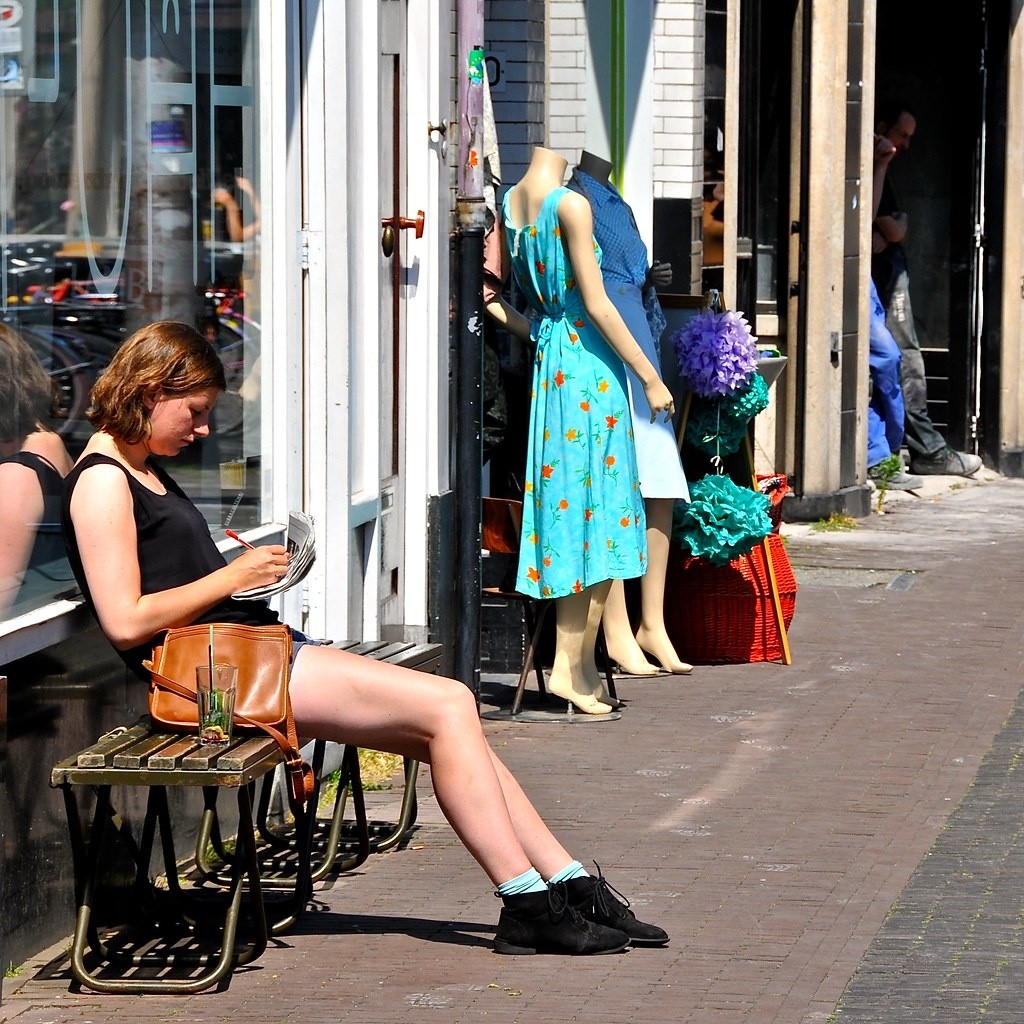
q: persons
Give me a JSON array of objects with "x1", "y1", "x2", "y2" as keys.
[
  {"x1": 875, "y1": 108, "x2": 982, "y2": 476},
  {"x1": 566, "y1": 151, "x2": 693, "y2": 676},
  {"x1": 61, "y1": 320, "x2": 670, "y2": 957},
  {"x1": 502, "y1": 147, "x2": 675, "y2": 715},
  {"x1": 0, "y1": 325, "x2": 73, "y2": 857}
]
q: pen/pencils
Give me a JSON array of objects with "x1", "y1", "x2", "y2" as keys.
[{"x1": 226, "y1": 529, "x2": 256, "y2": 552}]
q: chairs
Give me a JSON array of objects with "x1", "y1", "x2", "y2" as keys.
[{"x1": 479, "y1": 496, "x2": 618, "y2": 716}]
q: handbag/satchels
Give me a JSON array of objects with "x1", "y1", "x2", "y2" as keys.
[{"x1": 140, "y1": 621, "x2": 292, "y2": 732}]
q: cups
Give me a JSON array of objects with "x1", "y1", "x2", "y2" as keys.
[
  {"x1": 232, "y1": 458, "x2": 246, "y2": 489},
  {"x1": 196, "y1": 665, "x2": 238, "y2": 747},
  {"x1": 219, "y1": 460, "x2": 243, "y2": 489}
]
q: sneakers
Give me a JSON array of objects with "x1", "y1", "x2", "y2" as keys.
[
  {"x1": 911, "y1": 444, "x2": 982, "y2": 475},
  {"x1": 556, "y1": 862, "x2": 672, "y2": 944},
  {"x1": 491, "y1": 891, "x2": 630, "y2": 957},
  {"x1": 867, "y1": 466, "x2": 924, "y2": 490}
]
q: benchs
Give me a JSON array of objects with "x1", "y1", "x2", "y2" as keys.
[{"x1": 50, "y1": 640, "x2": 447, "y2": 993}]
